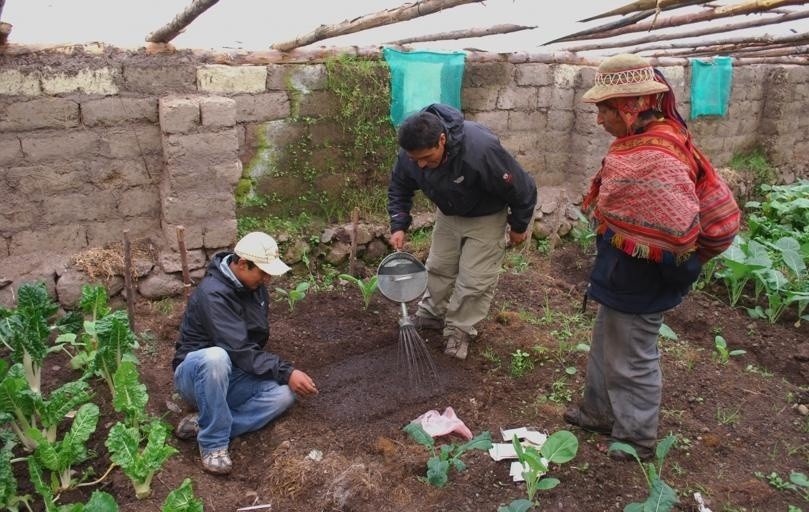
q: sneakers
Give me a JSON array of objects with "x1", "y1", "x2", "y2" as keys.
[
  {"x1": 198, "y1": 443, "x2": 232, "y2": 474},
  {"x1": 176, "y1": 413, "x2": 200, "y2": 439},
  {"x1": 410, "y1": 314, "x2": 468, "y2": 359},
  {"x1": 611, "y1": 446, "x2": 653, "y2": 462},
  {"x1": 565, "y1": 410, "x2": 610, "y2": 430}
]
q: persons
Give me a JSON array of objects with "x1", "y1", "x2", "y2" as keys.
[
  {"x1": 387, "y1": 112, "x2": 536, "y2": 361},
  {"x1": 563, "y1": 52, "x2": 742, "y2": 462},
  {"x1": 171, "y1": 231, "x2": 320, "y2": 475}
]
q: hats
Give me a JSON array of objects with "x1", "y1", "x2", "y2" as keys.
[
  {"x1": 583, "y1": 55, "x2": 670, "y2": 102},
  {"x1": 234, "y1": 232, "x2": 292, "y2": 276}
]
